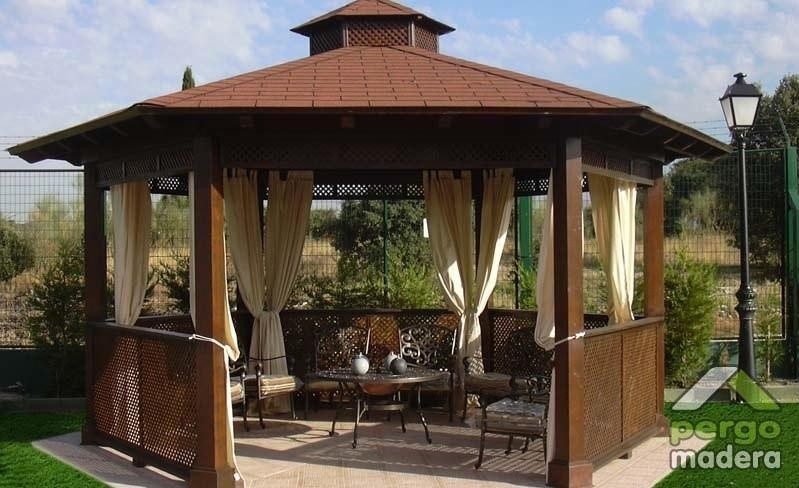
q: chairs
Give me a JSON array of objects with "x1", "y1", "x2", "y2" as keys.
[{"x1": 167, "y1": 324, "x2": 554, "y2": 469}]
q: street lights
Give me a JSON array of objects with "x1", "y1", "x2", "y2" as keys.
[{"x1": 719, "y1": 71, "x2": 763, "y2": 402}]
